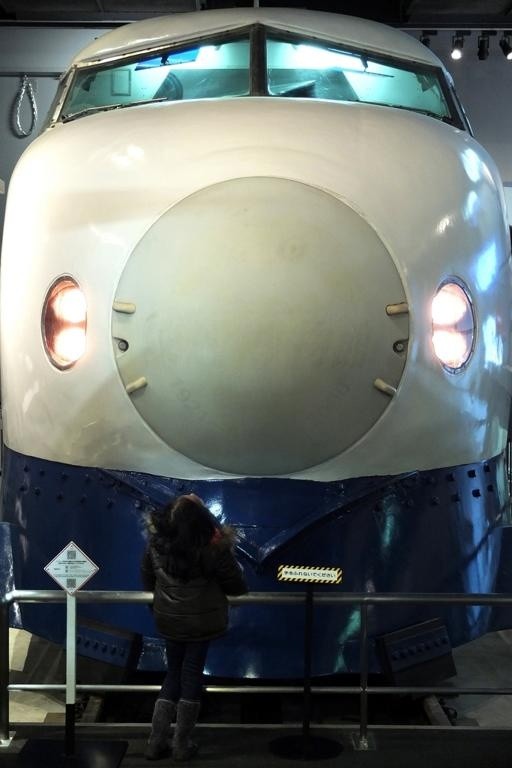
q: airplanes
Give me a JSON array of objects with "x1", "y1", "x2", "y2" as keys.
[{"x1": 0, "y1": 2, "x2": 511, "y2": 726}]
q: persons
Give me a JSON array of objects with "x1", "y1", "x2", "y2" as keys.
[{"x1": 141, "y1": 492, "x2": 250, "y2": 761}]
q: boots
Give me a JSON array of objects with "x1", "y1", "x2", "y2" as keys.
[
  {"x1": 174, "y1": 698, "x2": 200, "y2": 761},
  {"x1": 145, "y1": 699, "x2": 176, "y2": 758}
]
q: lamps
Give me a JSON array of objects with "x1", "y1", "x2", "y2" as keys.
[{"x1": 418, "y1": 28, "x2": 512, "y2": 62}]
